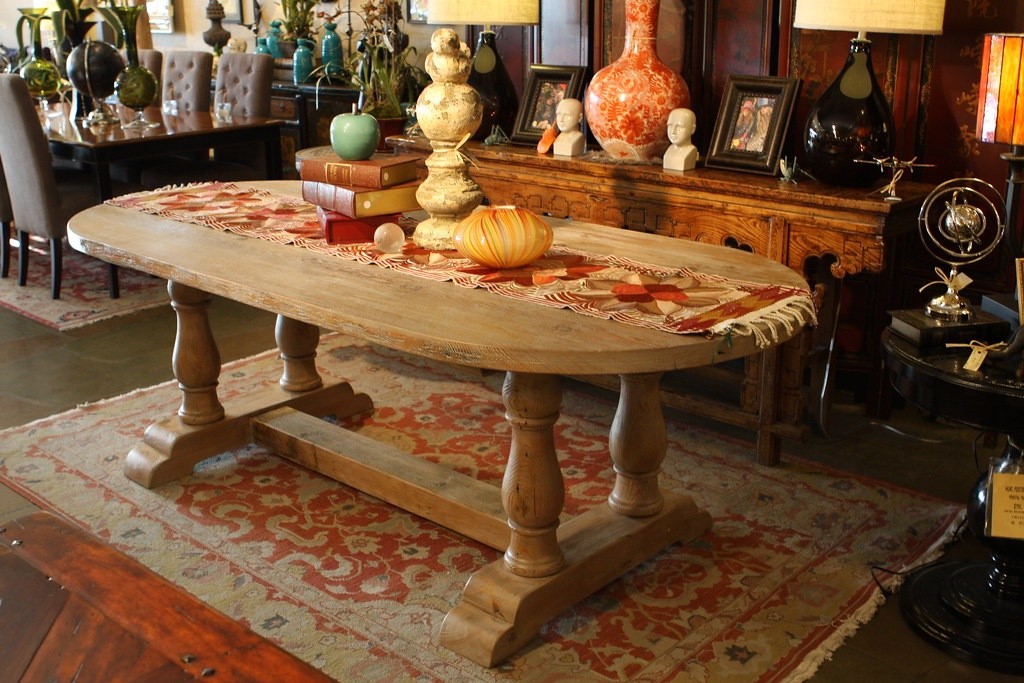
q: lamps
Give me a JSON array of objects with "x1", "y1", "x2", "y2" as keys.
[
  {"x1": 791, "y1": 0, "x2": 947, "y2": 192},
  {"x1": 427, "y1": 0, "x2": 540, "y2": 140},
  {"x1": 976, "y1": 34, "x2": 1024, "y2": 293}
]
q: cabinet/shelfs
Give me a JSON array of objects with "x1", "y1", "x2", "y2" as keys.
[{"x1": 210, "y1": 78, "x2": 360, "y2": 180}]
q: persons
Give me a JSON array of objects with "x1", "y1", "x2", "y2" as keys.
[
  {"x1": 535, "y1": 90, "x2": 557, "y2": 130},
  {"x1": 730, "y1": 99, "x2": 773, "y2": 151},
  {"x1": 552, "y1": 99, "x2": 586, "y2": 156},
  {"x1": 662, "y1": 107, "x2": 698, "y2": 171}
]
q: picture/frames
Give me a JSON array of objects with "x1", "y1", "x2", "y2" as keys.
[
  {"x1": 705, "y1": 74, "x2": 804, "y2": 178},
  {"x1": 218, "y1": 0, "x2": 244, "y2": 25},
  {"x1": 509, "y1": 63, "x2": 589, "y2": 151},
  {"x1": 406, "y1": 0, "x2": 456, "y2": 27}
]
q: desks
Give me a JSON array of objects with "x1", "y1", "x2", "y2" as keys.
[
  {"x1": 878, "y1": 303, "x2": 1024, "y2": 676},
  {"x1": 385, "y1": 129, "x2": 937, "y2": 466},
  {"x1": 67, "y1": 177, "x2": 816, "y2": 663}
]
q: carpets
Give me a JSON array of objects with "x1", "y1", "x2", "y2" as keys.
[
  {"x1": 0, "y1": 174, "x2": 171, "y2": 333},
  {"x1": 0, "y1": 336, "x2": 969, "y2": 683}
]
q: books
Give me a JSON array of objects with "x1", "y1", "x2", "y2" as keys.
[{"x1": 296, "y1": 148, "x2": 424, "y2": 245}]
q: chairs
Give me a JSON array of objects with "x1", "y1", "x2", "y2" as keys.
[{"x1": 0, "y1": 46, "x2": 274, "y2": 299}]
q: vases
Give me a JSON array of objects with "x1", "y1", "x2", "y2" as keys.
[
  {"x1": 268, "y1": 20, "x2": 286, "y2": 58},
  {"x1": 293, "y1": 38, "x2": 315, "y2": 86},
  {"x1": 17, "y1": 5, "x2": 61, "y2": 118},
  {"x1": 584, "y1": 0, "x2": 692, "y2": 165},
  {"x1": 93, "y1": 5, "x2": 127, "y2": 51},
  {"x1": 61, "y1": 20, "x2": 99, "y2": 122},
  {"x1": 320, "y1": 25, "x2": 343, "y2": 76},
  {"x1": 111, "y1": 3, "x2": 160, "y2": 130},
  {"x1": 255, "y1": 38, "x2": 272, "y2": 55}
]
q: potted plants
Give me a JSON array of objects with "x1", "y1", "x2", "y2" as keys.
[
  {"x1": 276, "y1": 0, "x2": 325, "y2": 57},
  {"x1": 307, "y1": 38, "x2": 427, "y2": 154}
]
